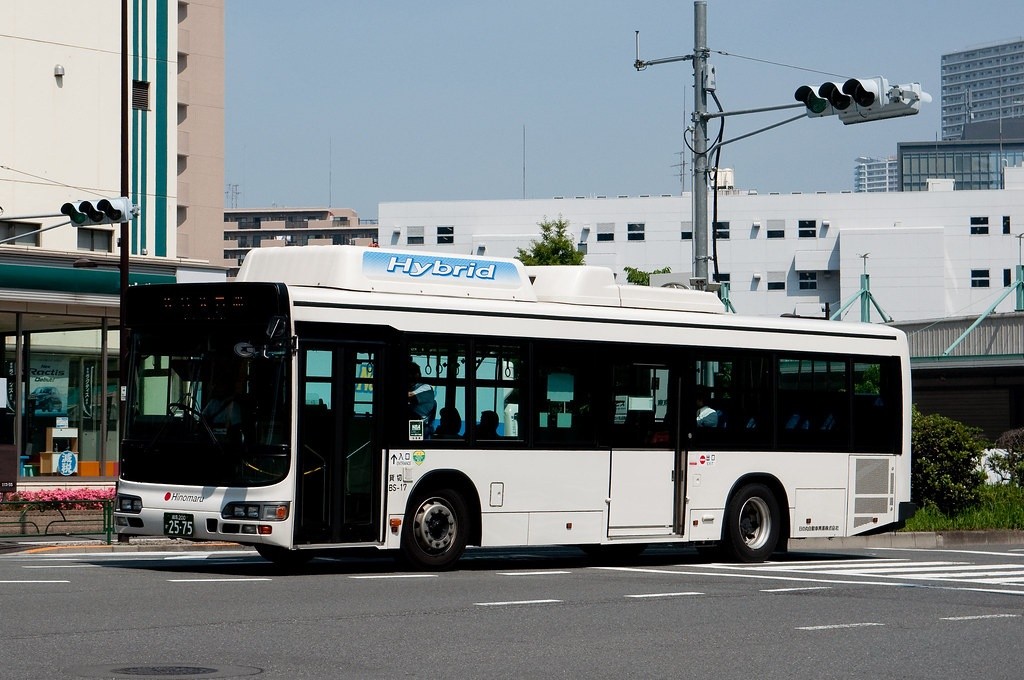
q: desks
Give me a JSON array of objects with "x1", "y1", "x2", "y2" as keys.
[
  {"x1": 19, "y1": 456, "x2": 29, "y2": 477},
  {"x1": 39, "y1": 452, "x2": 78, "y2": 477}
]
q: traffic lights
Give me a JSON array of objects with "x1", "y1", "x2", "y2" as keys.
[
  {"x1": 61, "y1": 196, "x2": 131, "y2": 228},
  {"x1": 792, "y1": 75, "x2": 889, "y2": 118},
  {"x1": 837, "y1": 81, "x2": 933, "y2": 125}
]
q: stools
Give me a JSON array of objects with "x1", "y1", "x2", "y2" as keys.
[{"x1": 24, "y1": 465, "x2": 34, "y2": 477}]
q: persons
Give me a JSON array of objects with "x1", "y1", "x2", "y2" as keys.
[
  {"x1": 202, "y1": 385, "x2": 233, "y2": 430},
  {"x1": 407, "y1": 361, "x2": 434, "y2": 419},
  {"x1": 696, "y1": 384, "x2": 718, "y2": 427},
  {"x1": 436, "y1": 408, "x2": 461, "y2": 437},
  {"x1": 475, "y1": 411, "x2": 499, "y2": 437}
]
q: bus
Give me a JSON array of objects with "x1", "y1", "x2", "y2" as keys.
[{"x1": 113, "y1": 241, "x2": 916, "y2": 574}]
